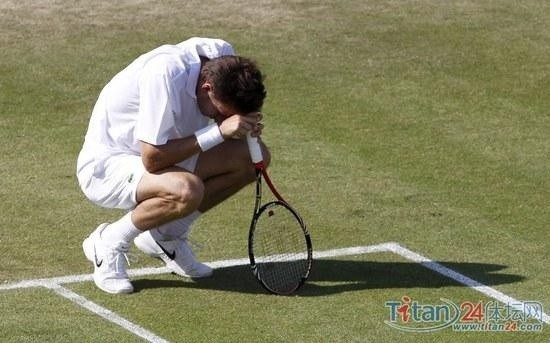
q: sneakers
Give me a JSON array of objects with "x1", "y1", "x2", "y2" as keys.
[
  {"x1": 83, "y1": 223, "x2": 134, "y2": 293},
  {"x1": 133, "y1": 229, "x2": 212, "y2": 277}
]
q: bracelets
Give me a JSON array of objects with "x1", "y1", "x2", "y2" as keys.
[{"x1": 193, "y1": 122, "x2": 225, "y2": 152}]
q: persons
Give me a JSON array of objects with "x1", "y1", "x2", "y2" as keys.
[{"x1": 75, "y1": 35, "x2": 268, "y2": 295}]
